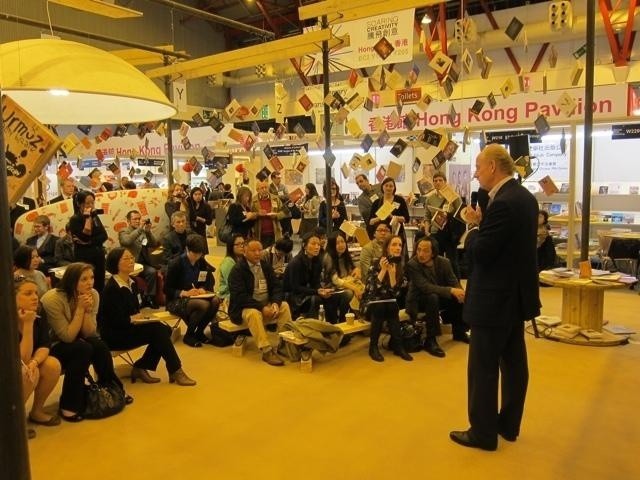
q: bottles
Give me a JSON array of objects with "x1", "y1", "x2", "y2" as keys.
[{"x1": 318, "y1": 305, "x2": 326, "y2": 323}]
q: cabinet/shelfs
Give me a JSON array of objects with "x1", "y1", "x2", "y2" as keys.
[{"x1": 537, "y1": 192, "x2": 640, "y2": 281}]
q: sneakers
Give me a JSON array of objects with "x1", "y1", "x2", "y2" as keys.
[
  {"x1": 184, "y1": 333, "x2": 212, "y2": 347},
  {"x1": 28, "y1": 412, "x2": 61, "y2": 426}
]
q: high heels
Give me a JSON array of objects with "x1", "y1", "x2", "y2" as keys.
[
  {"x1": 131, "y1": 364, "x2": 160, "y2": 383},
  {"x1": 169, "y1": 367, "x2": 196, "y2": 386}
]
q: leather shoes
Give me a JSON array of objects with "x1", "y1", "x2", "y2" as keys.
[
  {"x1": 59, "y1": 407, "x2": 82, "y2": 422},
  {"x1": 424, "y1": 337, "x2": 446, "y2": 357},
  {"x1": 262, "y1": 351, "x2": 283, "y2": 364},
  {"x1": 450, "y1": 430, "x2": 497, "y2": 450},
  {"x1": 454, "y1": 330, "x2": 470, "y2": 343},
  {"x1": 143, "y1": 294, "x2": 160, "y2": 309}
]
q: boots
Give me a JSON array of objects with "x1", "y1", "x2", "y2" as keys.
[
  {"x1": 370, "y1": 315, "x2": 385, "y2": 361},
  {"x1": 387, "y1": 315, "x2": 412, "y2": 360}
]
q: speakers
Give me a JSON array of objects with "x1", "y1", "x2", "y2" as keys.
[{"x1": 509, "y1": 135, "x2": 530, "y2": 161}]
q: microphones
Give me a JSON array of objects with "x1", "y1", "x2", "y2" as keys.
[{"x1": 471, "y1": 191, "x2": 479, "y2": 211}]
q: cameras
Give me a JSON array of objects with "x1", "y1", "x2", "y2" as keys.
[
  {"x1": 176, "y1": 191, "x2": 187, "y2": 198},
  {"x1": 388, "y1": 255, "x2": 402, "y2": 264},
  {"x1": 91, "y1": 208, "x2": 105, "y2": 218}
]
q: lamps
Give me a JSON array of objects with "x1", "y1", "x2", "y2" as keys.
[{"x1": 0, "y1": 1, "x2": 178, "y2": 127}]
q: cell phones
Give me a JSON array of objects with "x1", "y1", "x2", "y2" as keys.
[{"x1": 145, "y1": 219, "x2": 150, "y2": 225}]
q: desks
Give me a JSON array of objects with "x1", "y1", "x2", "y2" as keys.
[{"x1": 535, "y1": 267, "x2": 634, "y2": 347}]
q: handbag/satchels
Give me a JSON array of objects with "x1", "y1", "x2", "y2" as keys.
[
  {"x1": 84, "y1": 380, "x2": 127, "y2": 419},
  {"x1": 210, "y1": 318, "x2": 235, "y2": 346},
  {"x1": 360, "y1": 284, "x2": 399, "y2": 316},
  {"x1": 389, "y1": 320, "x2": 425, "y2": 353},
  {"x1": 219, "y1": 220, "x2": 242, "y2": 242}
]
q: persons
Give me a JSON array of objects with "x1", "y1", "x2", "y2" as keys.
[{"x1": 6, "y1": 143, "x2": 567, "y2": 454}]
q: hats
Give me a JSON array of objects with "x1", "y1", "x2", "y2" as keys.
[{"x1": 186, "y1": 234, "x2": 209, "y2": 256}]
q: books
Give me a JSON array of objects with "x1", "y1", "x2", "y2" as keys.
[
  {"x1": 536, "y1": 259, "x2": 639, "y2": 342},
  {"x1": 1, "y1": 92, "x2": 65, "y2": 207},
  {"x1": 60, "y1": 16, "x2": 639, "y2": 245}
]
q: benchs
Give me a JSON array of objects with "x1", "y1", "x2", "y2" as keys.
[
  {"x1": 110, "y1": 344, "x2": 142, "y2": 367},
  {"x1": 278, "y1": 311, "x2": 411, "y2": 346},
  {"x1": 130, "y1": 311, "x2": 183, "y2": 329},
  {"x1": 218, "y1": 319, "x2": 278, "y2": 333}
]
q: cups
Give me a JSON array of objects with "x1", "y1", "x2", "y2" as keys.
[{"x1": 345, "y1": 312, "x2": 356, "y2": 327}]
